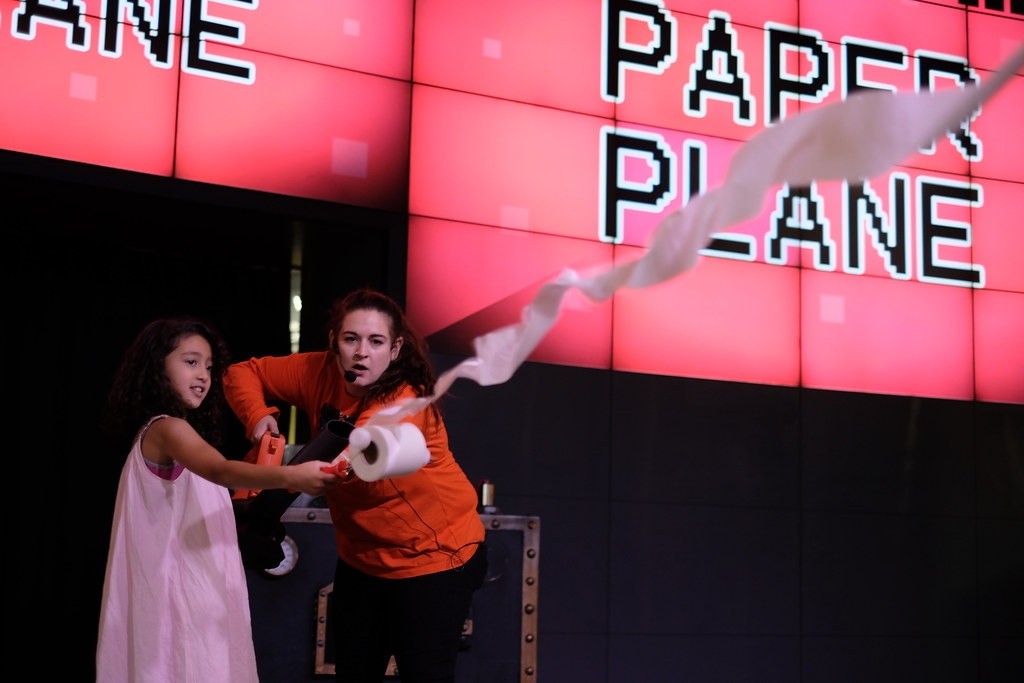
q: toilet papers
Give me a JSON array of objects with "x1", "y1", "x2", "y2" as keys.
[{"x1": 349, "y1": 45, "x2": 1024, "y2": 482}]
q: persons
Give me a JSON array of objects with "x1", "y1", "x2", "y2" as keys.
[
  {"x1": 95, "y1": 319, "x2": 342, "y2": 683},
  {"x1": 220, "y1": 290, "x2": 488, "y2": 683}
]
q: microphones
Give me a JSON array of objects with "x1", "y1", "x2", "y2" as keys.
[{"x1": 332, "y1": 337, "x2": 357, "y2": 383}]
